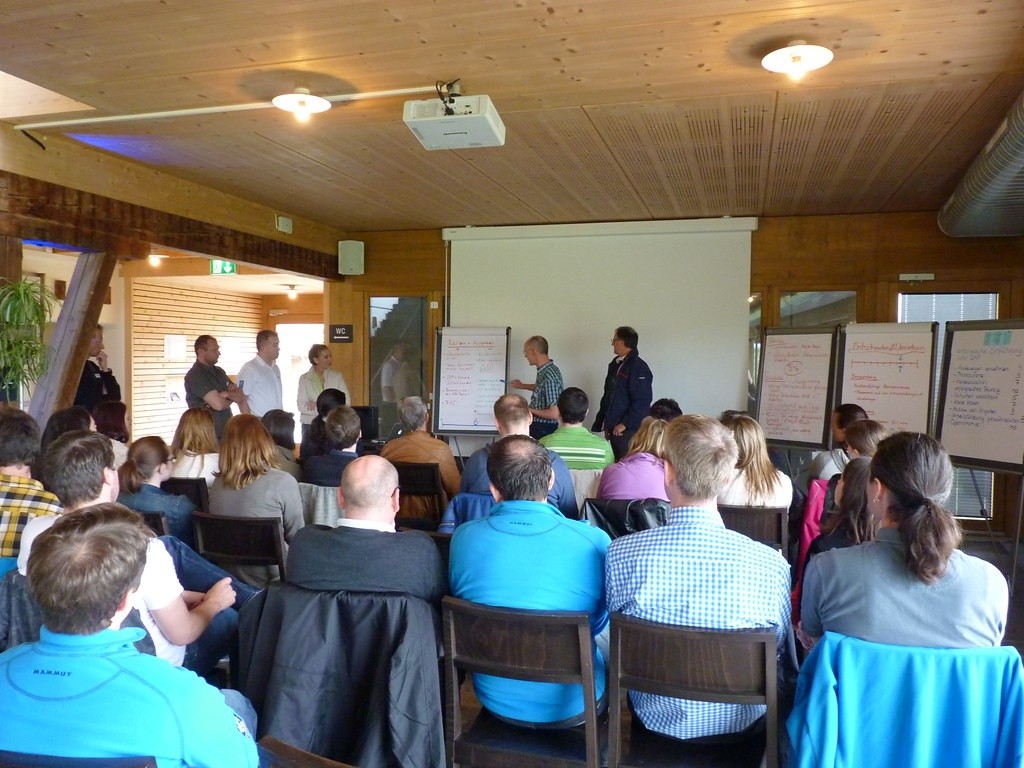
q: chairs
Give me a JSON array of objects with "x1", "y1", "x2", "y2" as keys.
[{"x1": 133, "y1": 454, "x2": 1024, "y2": 768}]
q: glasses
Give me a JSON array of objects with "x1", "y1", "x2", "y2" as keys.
[
  {"x1": 523, "y1": 351, "x2": 533, "y2": 354},
  {"x1": 166, "y1": 457, "x2": 176, "y2": 464},
  {"x1": 611, "y1": 339, "x2": 622, "y2": 342}
]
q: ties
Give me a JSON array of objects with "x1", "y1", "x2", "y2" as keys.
[{"x1": 612, "y1": 360, "x2": 622, "y2": 380}]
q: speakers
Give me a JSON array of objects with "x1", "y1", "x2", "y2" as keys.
[{"x1": 337, "y1": 240, "x2": 364, "y2": 275}]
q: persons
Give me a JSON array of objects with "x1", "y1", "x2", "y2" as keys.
[
  {"x1": 73, "y1": 324, "x2": 120, "y2": 412},
  {"x1": 379, "y1": 340, "x2": 407, "y2": 438},
  {"x1": 299, "y1": 344, "x2": 351, "y2": 425},
  {"x1": 392, "y1": 347, "x2": 423, "y2": 400},
  {"x1": 93, "y1": 400, "x2": 131, "y2": 468},
  {"x1": 0, "y1": 503, "x2": 258, "y2": 768},
  {"x1": 20, "y1": 430, "x2": 267, "y2": 677},
  {"x1": 169, "y1": 408, "x2": 220, "y2": 497},
  {"x1": 798, "y1": 432, "x2": 1008, "y2": 658},
  {"x1": 238, "y1": 330, "x2": 284, "y2": 422},
  {"x1": 308, "y1": 326, "x2": 886, "y2": 637},
  {"x1": 184, "y1": 336, "x2": 251, "y2": 437},
  {"x1": 261, "y1": 408, "x2": 304, "y2": 482},
  {"x1": 207, "y1": 415, "x2": 306, "y2": 587},
  {"x1": 300, "y1": 388, "x2": 347, "y2": 464},
  {"x1": 449, "y1": 435, "x2": 610, "y2": 732},
  {"x1": 41, "y1": 407, "x2": 98, "y2": 451},
  {"x1": 599, "y1": 416, "x2": 795, "y2": 768},
  {"x1": 115, "y1": 435, "x2": 198, "y2": 550},
  {"x1": 0, "y1": 400, "x2": 62, "y2": 557},
  {"x1": 336, "y1": 455, "x2": 466, "y2": 688}
]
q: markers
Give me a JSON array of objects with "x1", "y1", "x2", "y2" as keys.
[{"x1": 500, "y1": 380, "x2": 510, "y2": 384}]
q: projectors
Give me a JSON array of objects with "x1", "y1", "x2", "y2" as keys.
[{"x1": 402, "y1": 94, "x2": 506, "y2": 150}]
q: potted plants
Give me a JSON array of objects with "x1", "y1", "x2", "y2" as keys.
[{"x1": 0, "y1": 271, "x2": 65, "y2": 408}]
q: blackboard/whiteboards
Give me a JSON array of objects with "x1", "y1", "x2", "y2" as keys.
[
  {"x1": 838, "y1": 321, "x2": 940, "y2": 440},
  {"x1": 431, "y1": 326, "x2": 512, "y2": 436},
  {"x1": 756, "y1": 326, "x2": 841, "y2": 451},
  {"x1": 935, "y1": 318, "x2": 1024, "y2": 475}
]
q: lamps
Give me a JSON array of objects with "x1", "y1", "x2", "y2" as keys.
[
  {"x1": 760, "y1": 39, "x2": 834, "y2": 74},
  {"x1": 271, "y1": 88, "x2": 333, "y2": 120}
]
